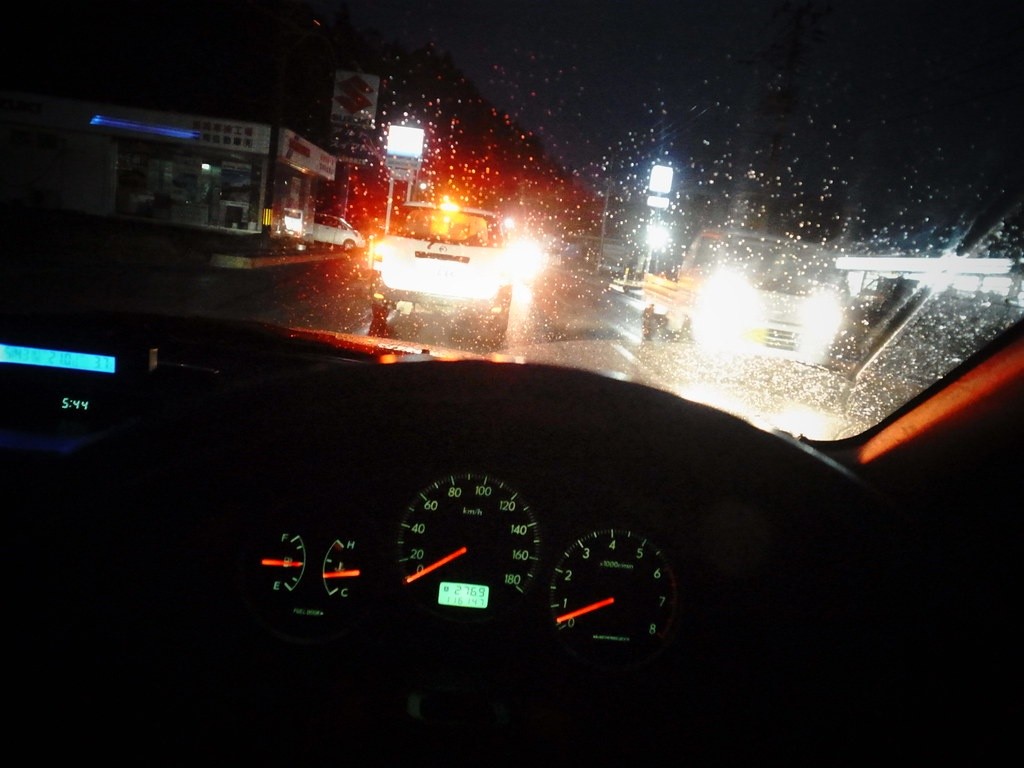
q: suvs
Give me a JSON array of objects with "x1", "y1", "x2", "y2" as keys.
[{"x1": 372, "y1": 199, "x2": 515, "y2": 335}]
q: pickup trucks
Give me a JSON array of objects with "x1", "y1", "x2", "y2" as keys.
[{"x1": 642, "y1": 229, "x2": 851, "y2": 373}]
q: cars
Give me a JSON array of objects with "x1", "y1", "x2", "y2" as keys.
[{"x1": 282, "y1": 207, "x2": 366, "y2": 253}]
